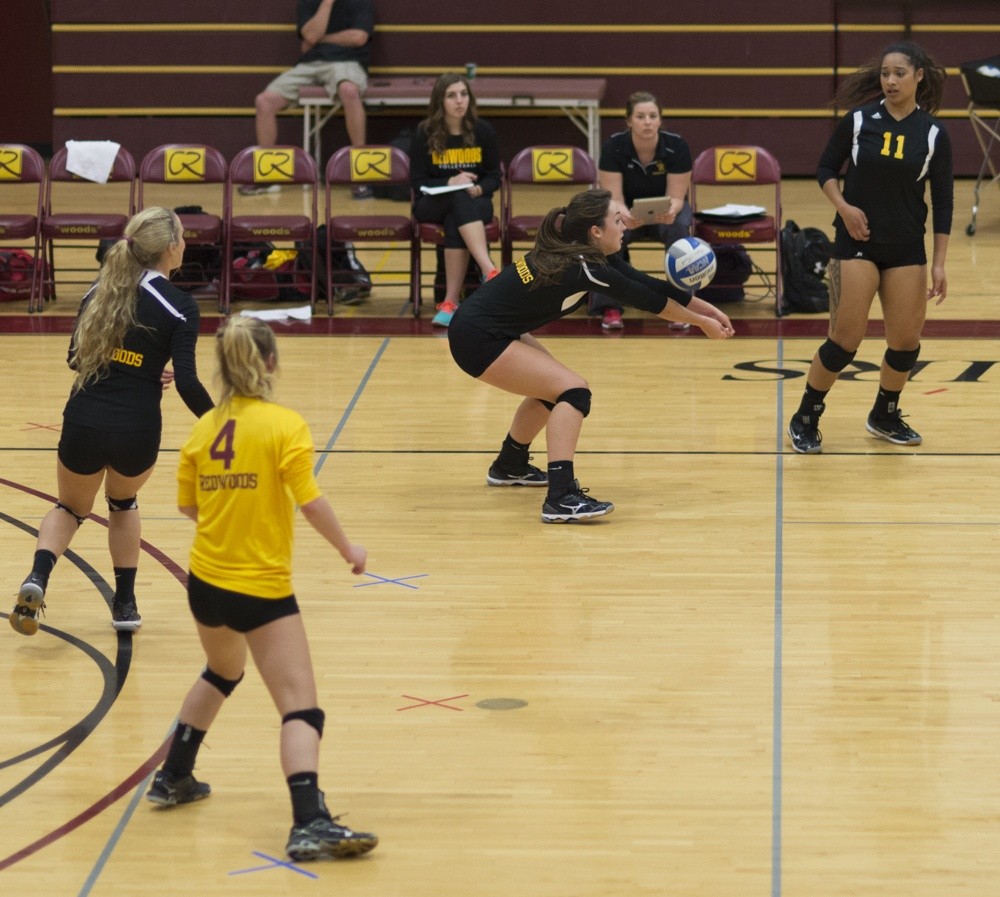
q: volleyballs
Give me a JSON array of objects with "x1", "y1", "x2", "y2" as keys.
[{"x1": 664, "y1": 237, "x2": 718, "y2": 292}]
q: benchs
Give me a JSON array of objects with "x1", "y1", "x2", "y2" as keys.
[{"x1": 39, "y1": 0, "x2": 1000, "y2": 176}]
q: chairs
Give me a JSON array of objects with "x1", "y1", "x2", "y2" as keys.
[
  {"x1": 37, "y1": 140, "x2": 135, "y2": 311},
  {"x1": 126, "y1": 143, "x2": 231, "y2": 311},
  {"x1": 504, "y1": 145, "x2": 596, "y2": 302},
  {"x1": 398, "y1": 154, "x2": 507, "y2": 304},
  {"x1": 672, "y1": 140, "x2": 793, "y2": 313},
  {"x1": 219, "y1": 143, "x2": 322, "y2": 316},
  {"x1": 0, "y1": 142, "x2": 47, "y2": 312},
  {"x1": 957, "y1": 48, "x2": 1000, "y2": 242},
  {"x1": 303, "y1": 145, "x2": 416, "y2": 313}
]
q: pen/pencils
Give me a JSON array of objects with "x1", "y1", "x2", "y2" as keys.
[{"x1": 456, "y1": 167, "x2": 477, "y2": 182}]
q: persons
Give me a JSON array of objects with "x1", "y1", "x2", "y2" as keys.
[
  {"x1": 448, "y1": 188, "x2": 734, "y2": 523},
  {"x1": 8, "y1": 207, "x2": 215, "y2": 637},
  {"x1": 410, "y1": 72, "x2": 501, "y2": 326},
  {"x1": 145, "y1": 315, "x2": 379, "y2": 859},
  {"x1": 599, "y1": 91, "x2": 694, "y2": 330},
  {"x1": 788, "y1": 40, "x2": 952, "y2": 454},
  {"x1": 237, "y1": 0, "x2": 376, "y2": 199}
]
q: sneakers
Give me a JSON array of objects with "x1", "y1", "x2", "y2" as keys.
[
  {"x1": 486, "y1": 452, "x2": 549, "y2": 486},
  {"x1": 285, "y1": 789, "x2": 378, "y2": 862},
  {"x1": 787, "y1": 403, "x2": 826, "y2": 453},
  {"x1": 146, "y1": 761, "x2": 211, "y2": 805},
  {"x1": 541, "y1": 478, "x2": 614, "y2": 523},
  {"x1": 866, "y1": 408, "x2": 922, "y2": 445}
]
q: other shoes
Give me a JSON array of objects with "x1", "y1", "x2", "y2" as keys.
[
  {"x1": 481, "y1": 269, "x2": 501, "y2": 283},
  {"x1": 353, "y1": 185, "x2": 372, "y2": 198},
  {"x1": 602, "y1": 308, "x2": 624, "y2": 329},
  {"x1": 239, "y1": 184, "x2": 281, "y2": 196},
  {"x1": 9, "y1": 573, "x2": 46, "y2": 636},
  {"x1": 111, "y1": 592, "x2": 142, "y2": 629},
  {"x1": 431, "y1": 299, "x2": 461, "y2": 326},
  {"x1": 669, "y1": 319, "x2": 690, "y2": 329}
]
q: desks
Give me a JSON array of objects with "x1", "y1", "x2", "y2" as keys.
[{"x1": 300, "y1": 73, "x2": 609, "y2": 175}]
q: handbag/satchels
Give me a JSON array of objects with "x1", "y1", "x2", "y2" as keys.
[
  {"x1": 229, "y1": 250, "x2": 320, "y2": 301},
  {"x1": 1, "y1": 250, "x2": 53, "y2": 300},
  {"x1": 698, "y1": 243, "x2": 751, "y2": 303}
]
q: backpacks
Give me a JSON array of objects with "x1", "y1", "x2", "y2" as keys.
[
  {"x1": 168, "y1": 204, "x2": 275, "y2": 290},
  {"x1": 780, "y1": 219, "x2": 833, "y2": 313},
  {"x1": 294, "y1": 225, "x2": 372, "y2": 300}
]
